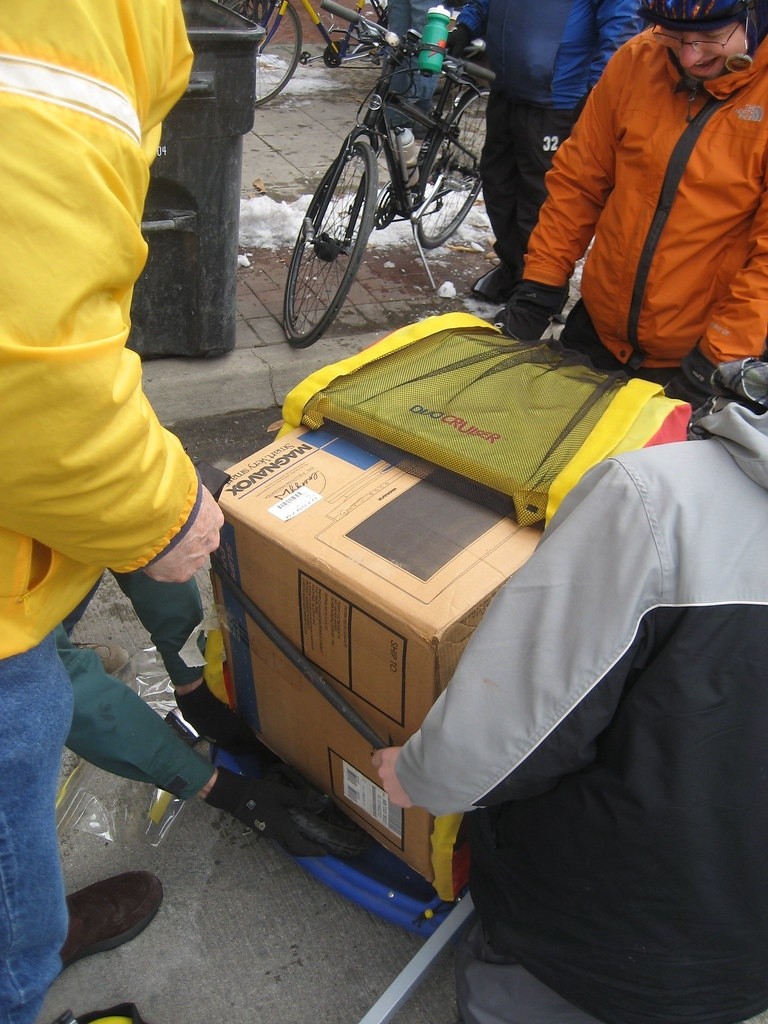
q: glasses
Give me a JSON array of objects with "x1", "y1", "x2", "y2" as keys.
[{"x1": 652, "y1": 20, "x2": 742, "y2": 56}]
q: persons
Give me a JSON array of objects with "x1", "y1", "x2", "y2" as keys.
[
  {"x1": 55, "y1": 568, "x2": 328, "y2": 857},
  {"x1": 447, "y1": 0, "x2": 649, "y2": 305},
  {"x1": 4, "y1": 0, "x2": 233, "y2": 1024},
  {"x1": 379, "y1": 3, "x2": 459, "y2": 140},
  {"x1": 498, "y1": 1, "x2": 768, "y2": 402},
  {"x1": 372, "y1": 359, "x2": 767, "y2": 1023}
]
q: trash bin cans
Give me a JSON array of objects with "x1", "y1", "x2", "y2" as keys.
[{"x1": 122, "y1": 0, "x2": 267, "y2": 358}]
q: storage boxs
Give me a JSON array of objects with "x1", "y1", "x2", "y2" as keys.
[{"x1": 206, "y1": 318, "x2": 691, "y2": 904}]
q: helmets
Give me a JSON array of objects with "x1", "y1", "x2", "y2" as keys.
[{"x1": 637, "y1": 0, "x2": 749, "y2": 31}]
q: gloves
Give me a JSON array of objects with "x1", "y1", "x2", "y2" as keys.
[
  {"x1": 446, "y1": 31, "x2": 470, "y2": 57},
  {"x1": 177, "y1": 688, "x2": 253, "y2": 745},
  {"x1": 665, "y1": 347, "x2": 719, "y2": 412},
  {"x1": 205, "y1": 767, "x2": 328, "y2": 856},
  {"x1": 571, "y1": 83, "x2": 593, "y2": 124},
  {"x1": 494, "y1": 280, "x2": 569, "y2": 341}
]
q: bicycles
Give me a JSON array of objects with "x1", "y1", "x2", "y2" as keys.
[
  {"x1": 283, "y1": 0, "x2": 497, "y2": 349},
  {"x1": 223, "y1": 0, "x2": 460, "y2": 95}
]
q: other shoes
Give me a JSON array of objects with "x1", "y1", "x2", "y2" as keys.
[
  {"x1": 413, "y1": 121, "x2": 460, "y2": 139},
  {"x1": 73, "y1": 642, "x2": 129, "y2": 675},
  {"x1": 390, "y1": 117, "x2": 414, "y2": 128},
  {"x1": 473, "y1": 261, "x2": 523, "y2": 302},
  {"x1": 59, "y1": 870, "x2": 163, "y2": 968}
]
